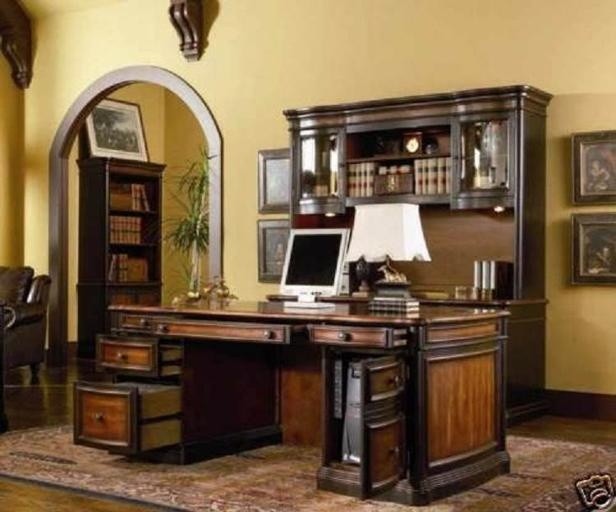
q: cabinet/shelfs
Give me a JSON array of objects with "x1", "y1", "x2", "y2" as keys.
[
  {"x1": 75, "y1": 161, "x2": 167, "y2": 380},
  {"x1": 258, "y1": 85, "x2": 552, "y2": 427}
]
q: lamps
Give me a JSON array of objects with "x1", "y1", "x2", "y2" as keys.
[{"x1": 343, "y1": 202, "x2": 432, "y2": 316}]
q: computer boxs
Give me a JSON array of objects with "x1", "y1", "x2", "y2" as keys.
[{"x1": 341, "y1": 358, "x2": 377, "y2": 465}]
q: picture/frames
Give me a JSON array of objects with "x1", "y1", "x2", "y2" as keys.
[
  {"x1": 257, "y1": 220, "x2": 292, "y2": 284},
  {"x1": 568, "y1": 132, "x2": 615, "y2": 286},
  {"x1": 256, "y1": 150, "x2": 293, "y2": 213},
  {"x1": 84, "y1": 98, "x2": 150, "y2": 162}
]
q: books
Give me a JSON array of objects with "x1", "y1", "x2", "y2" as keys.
[
  {"x1": 473, "y1": 259, "x2": 514, "y2": 301},
  {"x1": 108, "y1": 182, "x2": 158, "y2": 305},
  {"x1": 414, "y1": 157, "x2": 452, "y2": 195},
  {"x1": 348, "y1": 162, "x2": 375, "y2": 197}
]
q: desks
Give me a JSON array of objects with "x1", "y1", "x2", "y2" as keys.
[{"x1": 68, "y1": 297, "x2": 510, "y2": 507}]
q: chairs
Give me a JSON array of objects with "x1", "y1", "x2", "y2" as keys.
[{"x1": 0, "y1": 269, "x2": 51, "y2": 387}]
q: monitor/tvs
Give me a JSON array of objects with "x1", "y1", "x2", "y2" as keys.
[{"x1": 279, "y1": 228, "x2": 351, "y2": 308}]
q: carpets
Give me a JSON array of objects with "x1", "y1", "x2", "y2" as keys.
[{"x1": 0, "y1": 426, "x2": 616, "y2": 512}]
